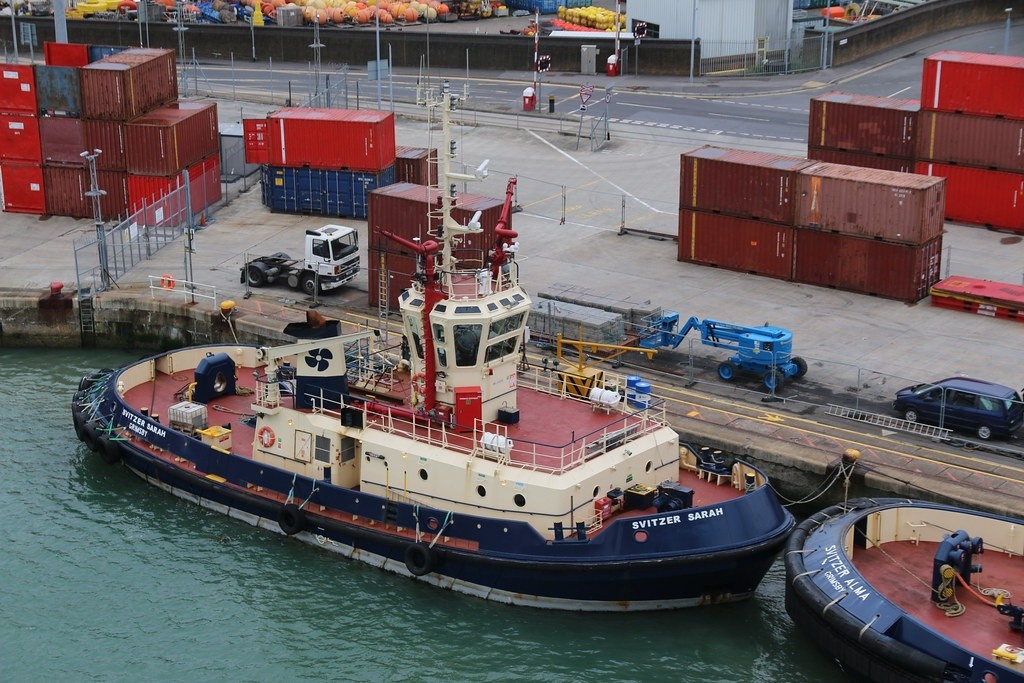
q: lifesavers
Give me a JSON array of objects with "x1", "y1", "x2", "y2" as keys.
[
  {"x1": 160, "y1": 273, "x2": 175, "y2": 290},
  {"x1": 277, "y1": 503, "x2": 306, "y2": 536},
  {"x1": 412, "y1": 373, "x2": 426, "y2": 394},
  {"x1": 258, "y1": 426, "x2": 275, "y2": 448},
  {"x1": 402, "y1": 541, "x2": 435, "y2": 576},
  {"x1": 71, "y1": 366, "x2": 120, "y2": 463}
]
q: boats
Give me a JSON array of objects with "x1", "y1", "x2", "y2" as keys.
[
  {"x1": 71, "y1": 74, "x2": 794, "y2": 612},
  {"x1": 785, "y1": 496, "x2": 1024, "y2": 683}
]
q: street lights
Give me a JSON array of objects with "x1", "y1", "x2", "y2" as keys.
[{"x1": 1005, "y1": 8, "x2": 1013, "y2": 56}]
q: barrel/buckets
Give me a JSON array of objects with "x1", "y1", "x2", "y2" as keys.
[
  {"x1": 627, "y1": 375, "x2": 642, "y2": 403},
  {"x1": 636, "y1": 382, "x2": 652, "y2": 409}
]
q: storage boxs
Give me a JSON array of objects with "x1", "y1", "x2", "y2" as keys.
[
  {"x1": 676, "y1": 49, "x2": 1024, "y2": 305},
  {"x1": 0, "y1": 39, "x2": 515, "y2": 315}
]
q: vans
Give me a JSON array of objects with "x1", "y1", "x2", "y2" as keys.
[{"x1": 895, "y1": 377, "x2": 1024, "y2": 440}]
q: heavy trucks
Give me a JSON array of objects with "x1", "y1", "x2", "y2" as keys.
[{"x1": 240, "y1": 224, "x2": 361, "y2": 296}]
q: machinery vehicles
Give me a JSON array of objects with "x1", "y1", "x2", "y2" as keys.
[{"x1": 637, "y1": 309, "x2": 808, "y2": 393}]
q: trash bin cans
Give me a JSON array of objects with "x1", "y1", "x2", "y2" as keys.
[
  {"x1": 607, "y1": 55, "x2": 619, "y2": 76},
  {"x1": 523, "y1": 88, "x2": 534, "y2": 111}
]
